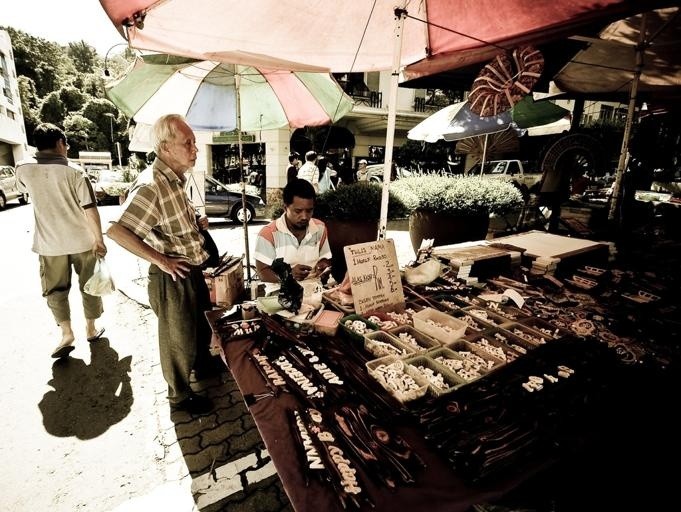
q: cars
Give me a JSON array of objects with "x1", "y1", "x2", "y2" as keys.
[
  {"x1": 0, "y1": 164, "x2": 29, "y2": 211},
  {"x1": 81, "y1": 167, "x2": 124, "y2": 206},
  {"x1": 355, "y1": 159, "x2": 412, "y2": 189},
  {"x1": 204, "y1": 174, "x2": 261, "y2": 226}
]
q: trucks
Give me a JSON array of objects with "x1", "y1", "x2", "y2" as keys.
[{"x1": 468, "y1": 159, "x2": 543, "y2": 192}]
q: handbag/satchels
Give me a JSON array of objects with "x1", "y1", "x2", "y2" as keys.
[{"x1": 405, "y1": 260, "x2": 441, "y2": 286}]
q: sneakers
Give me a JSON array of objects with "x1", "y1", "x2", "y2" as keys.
[
  {"x1": 195, "y1": 354, "x2": 226, "y2": 379},
  {"x1": 171, "y1": 394, "x2": 215, "y2": 413}
]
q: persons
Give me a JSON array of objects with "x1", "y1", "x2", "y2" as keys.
[
  {"x1": 16, "y1": 123, "x2": 107, "y2": 358},
  {"x1": 254, "y1": 178, "x2": 333, "y2": 281},
  {"x1": 287, "y1": 147, "x2": 368, "y2": 199},
  {"x1": 107, "y1": 115, "x2": 224, "y2": 415}
]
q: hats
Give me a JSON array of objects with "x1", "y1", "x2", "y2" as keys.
[{"x1": 357, "y1": 160, "x2": 367, "y2": 164}]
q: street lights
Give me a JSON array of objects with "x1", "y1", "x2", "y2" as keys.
[{"x1": 98, "y1": 42, "x2": 143, "y2": 80}]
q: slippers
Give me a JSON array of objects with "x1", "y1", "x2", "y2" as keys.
[
  {"x1": 87, "y1": 329, "x2": 105, "y2": 341},
  {"x1": 51, "y1": 345, "x2": 75, "y2": 357}
]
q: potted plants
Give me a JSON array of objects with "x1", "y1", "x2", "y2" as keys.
[{"x1": 268, "y1": 169, "x2": 532, "y2": 256}]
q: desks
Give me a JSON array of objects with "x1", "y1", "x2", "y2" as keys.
[{"x1": 202, "y1": 257, "x2": 678, "y2": 509}]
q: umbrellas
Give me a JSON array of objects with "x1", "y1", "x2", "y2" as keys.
[
  {"x1": 101, "y1": 0, "x2": 622, "y2": 242},
  {"x1": 105, "y1": 53, "x2": 355, "y2": 283},
  {"x1": 398, "y1": 7, "x2": 681, "y2": 221},
  {"x1": 407, "y1": 93, "x2": 570, "y2": 175}
]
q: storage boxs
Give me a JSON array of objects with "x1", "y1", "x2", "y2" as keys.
[{"x1": 200, "y1": 253, "x2": 245, "y2": 307}]
